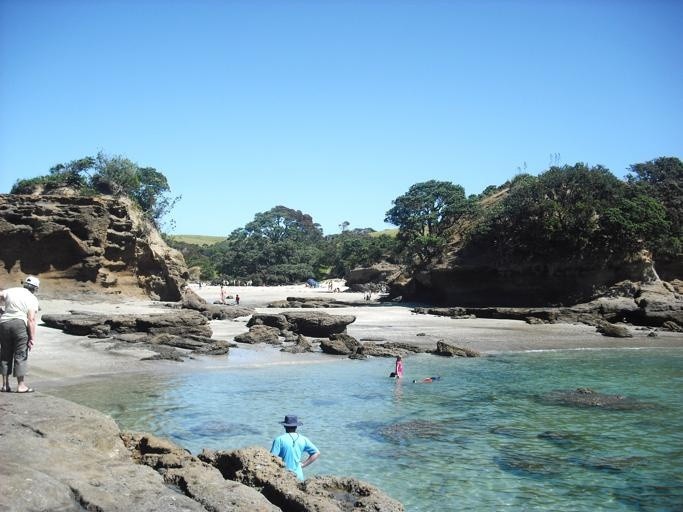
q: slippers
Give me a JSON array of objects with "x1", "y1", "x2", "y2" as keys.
[
  {"x1": 2, "y1": 386, "x2": 11, "y2": 391},
  {"x1": 17, "y1": 387, "x2": 34, "y2": 393}
]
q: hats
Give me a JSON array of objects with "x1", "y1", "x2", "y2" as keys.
[{"x1": 279, "y1": 415, "x2": 303, "y2": 427}]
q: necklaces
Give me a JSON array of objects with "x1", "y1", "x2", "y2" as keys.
[{"x1": 289, "y1": 432, "x2": 299, "y2": 448}]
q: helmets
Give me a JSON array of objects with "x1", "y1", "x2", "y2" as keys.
[{"x1": 25, "y1": 276, "x2": 40, "y2": 287}]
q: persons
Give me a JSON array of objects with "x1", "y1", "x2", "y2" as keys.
[
  {"x1": 220, "y1": 286, "x2": 227, "y2": 305},
  {"x1": 270, "y1": 415, "x2": 320, "y2": 480},
  {"x1": 0, "y1": 276, "x2": 40, "y2": 393},
  {"x1": 236, "y1": 294, "x2": 240, "y2": 304},
  {"x1": 395, "y1": 354, "x2": 403, "y2": 384},
  {"x1": 412, "y1": 376, "x2": 443, "y2": 383}
]
